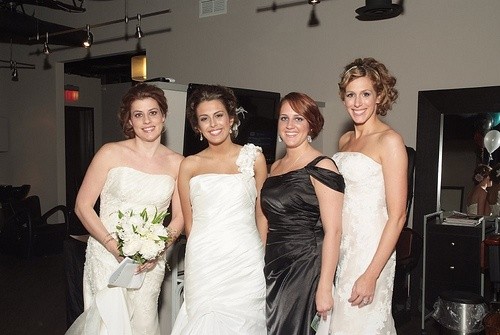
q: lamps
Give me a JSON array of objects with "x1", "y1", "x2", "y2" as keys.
[
  {"x1": 308, "y1": 0, "x2": 319, "y2": 26},
  {"x1": 42, "y1": 31, "x2": 50, "y2": 54},
  {"x1": 12, "y1": 60, "x2": 18, "y2": 80},
  {"x1": 82, "y1": 24, "x2": 93, "y2": 46},
  {"x1": 132, "y1": 53, "x2": 147, "y2": 81},
  {"x1": 136, "y1": 12, "x2": 143, "y2": 38}
]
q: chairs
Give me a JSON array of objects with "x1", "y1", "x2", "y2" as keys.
[{"x1": 0, "y1": 191, "x2": 71, "y2": 254}]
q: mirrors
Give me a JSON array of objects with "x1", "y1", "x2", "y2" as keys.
[{"x1": 440, "y1": 110, "x2": 500, "y2": 220}]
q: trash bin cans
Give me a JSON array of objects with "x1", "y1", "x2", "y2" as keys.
[{"x1": 437, "y1": 292, "x2": 485, "y2": 335}]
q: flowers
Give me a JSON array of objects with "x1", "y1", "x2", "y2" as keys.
[
  {"x1": 111, "y1": 206, "x2": 173, "y2": 270},
  {"x1": 475, "y1": 173, "x2": 484, "y2": 182}
]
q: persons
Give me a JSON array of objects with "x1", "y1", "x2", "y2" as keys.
[
  {"x1": 170, "y1": 85, "x2": 267, "y2": 335},
  {"x1": 314, "y1": 57, "x2": 409, "y2": 334},
  {"x1": 257, "y1": 92, "x2": 346, "y2": 335},
  {"x1": 65, "y1": 85, "x2": 183, "y2": 335}
]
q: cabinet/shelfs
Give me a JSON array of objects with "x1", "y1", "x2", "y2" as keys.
[{"x1": 422, "y1": 211, "x2": 500, "y2": 330}]
